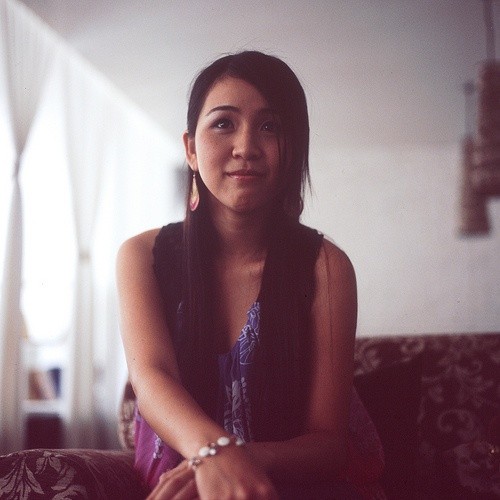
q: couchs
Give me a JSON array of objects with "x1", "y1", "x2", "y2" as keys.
[{"x1": 1, "y1": 333, "x2": 499, "y2": 500}]
